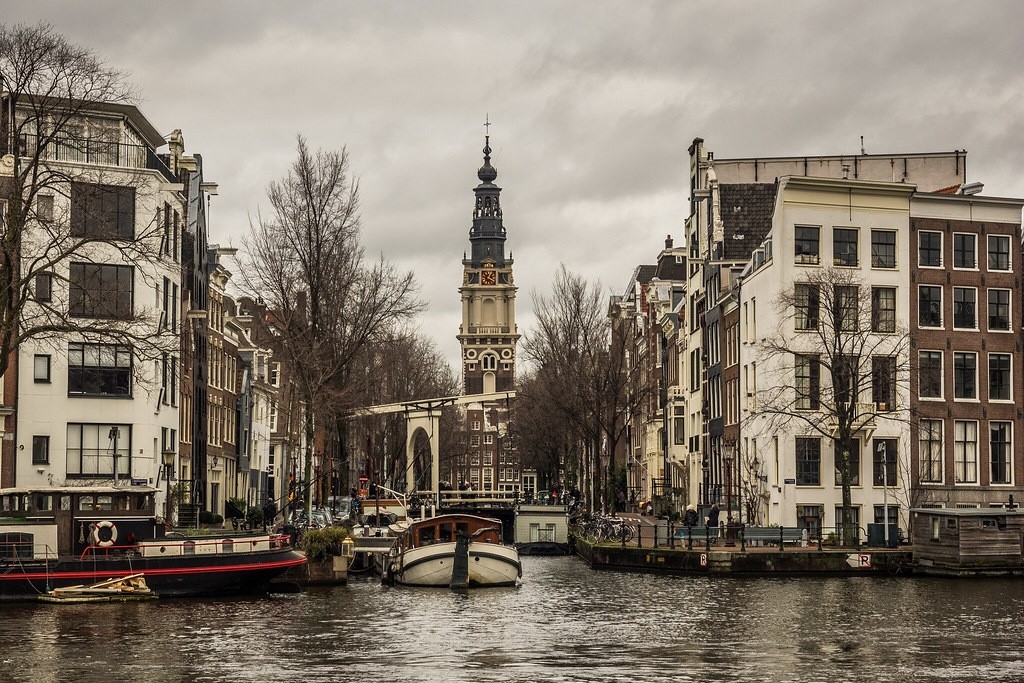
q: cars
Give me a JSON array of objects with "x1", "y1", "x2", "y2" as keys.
[{"x1": 288, "y1": 496, "x2": 354, "y2": 531}]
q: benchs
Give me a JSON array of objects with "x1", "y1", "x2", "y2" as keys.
[
  {"x1": 744, "y1": 528, "x2": 802, "y2": 547},
  {"x1": 674, "y1": 528, "x2": 719, "y2": 546}
]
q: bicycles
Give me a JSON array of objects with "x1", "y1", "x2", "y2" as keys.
[{"x1": 570, "y1": 501, "x2": 636, "y2": 544}]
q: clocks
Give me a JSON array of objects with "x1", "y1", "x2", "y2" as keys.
[{"x1": 481, "y1": 271, "x2": 496, "y2": 285}]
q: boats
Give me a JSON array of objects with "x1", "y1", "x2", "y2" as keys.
[
  {"x1": 37, "y1": 572, "x2": 160, "y2": 605},
  {"x1": 384, "y1": 504, "x2": 524, "y2": 588},
  {"x1": 910, "y1": 494, "x2": 1024, "y2": 578},
  {"x1": 0, "y1": 484, "x2": 312, "y2": 599}
]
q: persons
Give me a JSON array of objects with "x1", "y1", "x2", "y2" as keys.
[
  {"x1": 707, "y1": 503, "x2": 720, "y2": 544},
  {"x1": 683, "y1": 504, "x2": 699, "y2": 542}
]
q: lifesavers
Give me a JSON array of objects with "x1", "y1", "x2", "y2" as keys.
[{"x1": 94, "y1": 520, "x2": 118, "y2": 548}]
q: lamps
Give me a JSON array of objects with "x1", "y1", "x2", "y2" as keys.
[
  {"x1": 704, "y1": 455, "x2": 709, "y2": 468},
  {"x1": 211, "y1": 455, "x2": 218, "y2": 469},
  {"x1": 752, "y1": 458, "x2": 767, "y2": 482},
  {"x1": 341, "y1": 535, "x2": 355, "y2": 558},
  {"x1": 641, "y1": 475, "x2": 645, "y2": 481}
]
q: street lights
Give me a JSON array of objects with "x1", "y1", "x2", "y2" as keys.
[
  {"x1": 721, "y1": 439, "x2": 736, "y2": 547},
  {"x1": 877, "y1": 441, "x2": 891, "y2": 549}
]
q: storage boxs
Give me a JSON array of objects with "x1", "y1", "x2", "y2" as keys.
[{"x1": 879, "y1": 402, "x2": 886, "y2": 410}]
